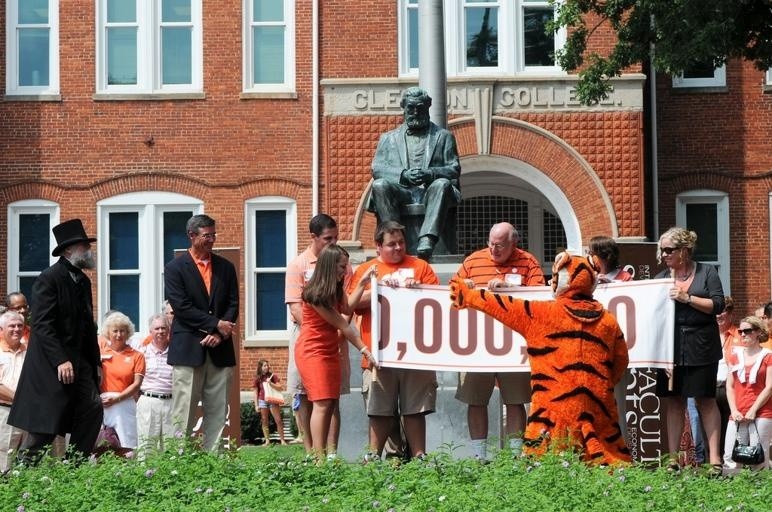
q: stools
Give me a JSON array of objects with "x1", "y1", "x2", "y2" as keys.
[{"x1": 139, "y1": 392, "x2": 171, "y2": 400}]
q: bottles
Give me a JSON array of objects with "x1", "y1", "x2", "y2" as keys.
[{"x1": 52, "y1": 219, "x2": 96, "y2": 257}]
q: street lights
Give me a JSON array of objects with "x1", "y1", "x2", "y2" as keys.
[
  {"x1": 732, "y1": 440, "x2": 764, "y2": 465},
  {"x1": 262, "y1": 382, "x2": 284, "y2": 405}
]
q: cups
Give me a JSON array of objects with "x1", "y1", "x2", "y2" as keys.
[
  {"x1": 660, "y1": 247, "x2": 679, "y2": 254},
  {"x1": 739, "y1": 328, "x2": 758, "y2": 334}
]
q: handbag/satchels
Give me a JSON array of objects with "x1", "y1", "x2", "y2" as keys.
[{"x1": 359, "y1": 345, "x2": 368, "y2": 355}]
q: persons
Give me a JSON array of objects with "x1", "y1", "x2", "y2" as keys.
[
  {"x1": 163, "y1": 214, "x2": 238, "y2": 459},
  {"x1": 293, "y1": 242, "x2": 380, "y2": 461},
  {"x1": 0, "y1": 291, "x2": 174, "y2": 462},
  {"x1": 253, "y1": 359, "x2": 288, "y2": 446},
  {"x1": 348, "y1": 220, "x2": 441, "y2": 463},
  {"x1": 6, "y1": 217, "x2": 104, "y2": 465},
  {"x1": 448, "y1": 221, "x2": 547, "y2": 460},
  {"x1": 680, "y1": 295, "x2": 772, "y2": 475},
  {"x1": 289, "y1": 394, "x2": 305, "y2": 445},
  {"x1": 369, "y1": 86, "x2": 464, "y2": 260},
  {"x1": 287, "y1": 214, "x2": 354, "y2": 459},
  {"x1": 585, "y1": 235, "x2": 633, "y2": 447},
  {"x1": 652, "y1": 227, "x2": 726, "y2": 479}
]
